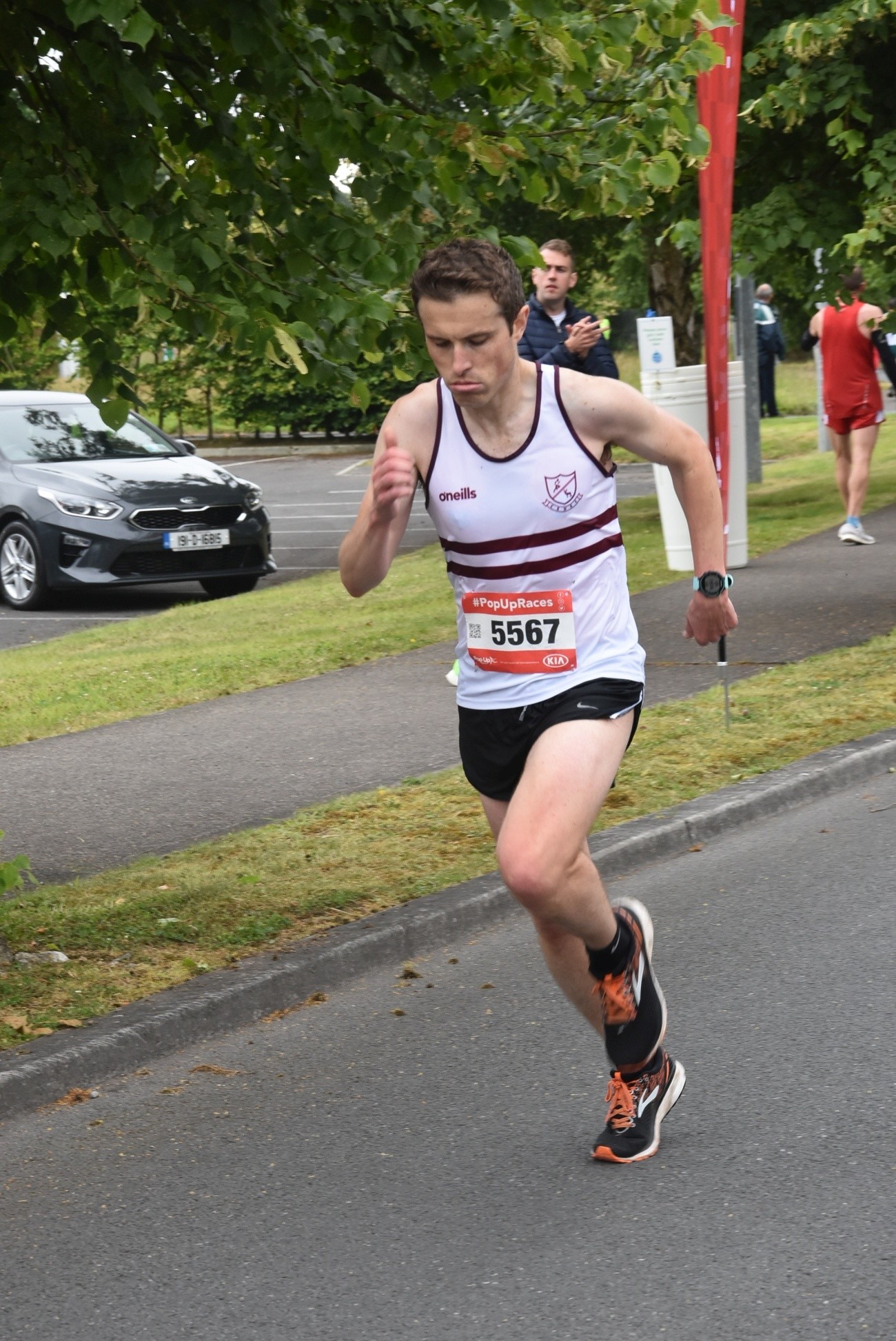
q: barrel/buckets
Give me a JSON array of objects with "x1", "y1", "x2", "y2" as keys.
[{"x1": 640, "y1": 360, "x2": 749, "y2": 570}]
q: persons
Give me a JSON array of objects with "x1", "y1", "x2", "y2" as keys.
[
  {"x1": 515, "y1": 238, "x2": 622, "y2": 382},
  {"x1": 336, "y1": 234, "x2": 741, "y2": 1166},
  {"x1": 801, "y1": 265, "x2": 896, "y2": 544},
  {"x1": 753, "y1": 281, "x2": 791, "y2": 418}
]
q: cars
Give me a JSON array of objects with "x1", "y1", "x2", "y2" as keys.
[{"x1": 0, "y1": 389, "x2": 278, "y2": 612}]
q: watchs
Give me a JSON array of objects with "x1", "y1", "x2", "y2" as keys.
[{"x1": 691, "y1": 570, "x2": 733, "y2": 600}]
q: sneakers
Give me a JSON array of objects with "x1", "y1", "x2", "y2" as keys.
[
  {"x1": 589, "y1": 896, "x2": 667, "y2": 1072},
  {"x1": 837, "y1": 523, "x2": 876, "y2": 543},
  {"x1": 589, "y1": 1047, "x2": 686, "y2": 1162}
]
